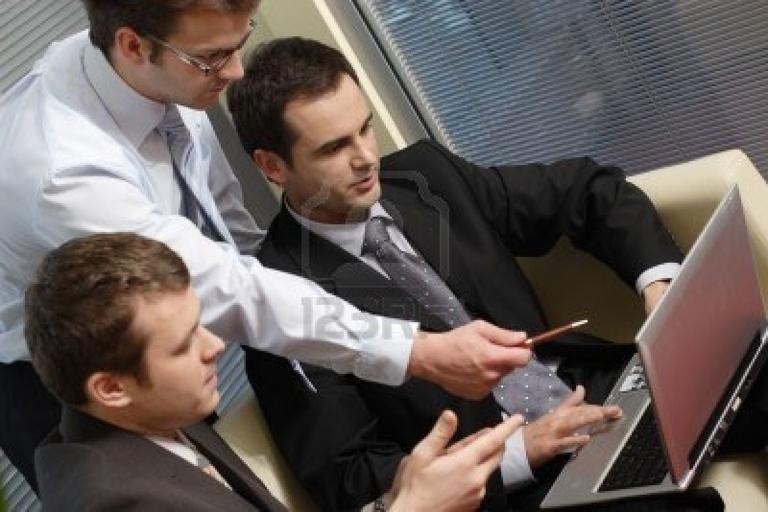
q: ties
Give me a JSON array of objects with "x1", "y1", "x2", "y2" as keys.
[
  {"x1": 157, "y1": 105, "x2": 319, "y2": 394},
  {"x1": 363, "y1": 218, "x2": 594, "y2": 455}
]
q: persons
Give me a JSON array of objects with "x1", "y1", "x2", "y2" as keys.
[
  {"x1": 0, "y1": 0, "x2": 534, "y2": 502},
  {"x1": 23, "y1": 231, "x2": 526, "y2": 512},
  {"x1": 225, "y1": 36, "x2": 766, "y2": 512}
]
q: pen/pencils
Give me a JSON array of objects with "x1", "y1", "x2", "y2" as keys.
[{"x1": 519, "y1": 318, "x2": 589, "y2": 349}]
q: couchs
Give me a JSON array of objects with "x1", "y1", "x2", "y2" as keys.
[{"x1": 212, "y1": 149, "x2": 768, "y2": 512}]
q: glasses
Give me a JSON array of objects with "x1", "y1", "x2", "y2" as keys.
[{"x1": 138, "y1": 17, "x2": 259, "y2": 77}]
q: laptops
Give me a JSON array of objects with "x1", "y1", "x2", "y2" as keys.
[{"x1": 537, "y1": 184, "x2": 767, "y2": 512}]
